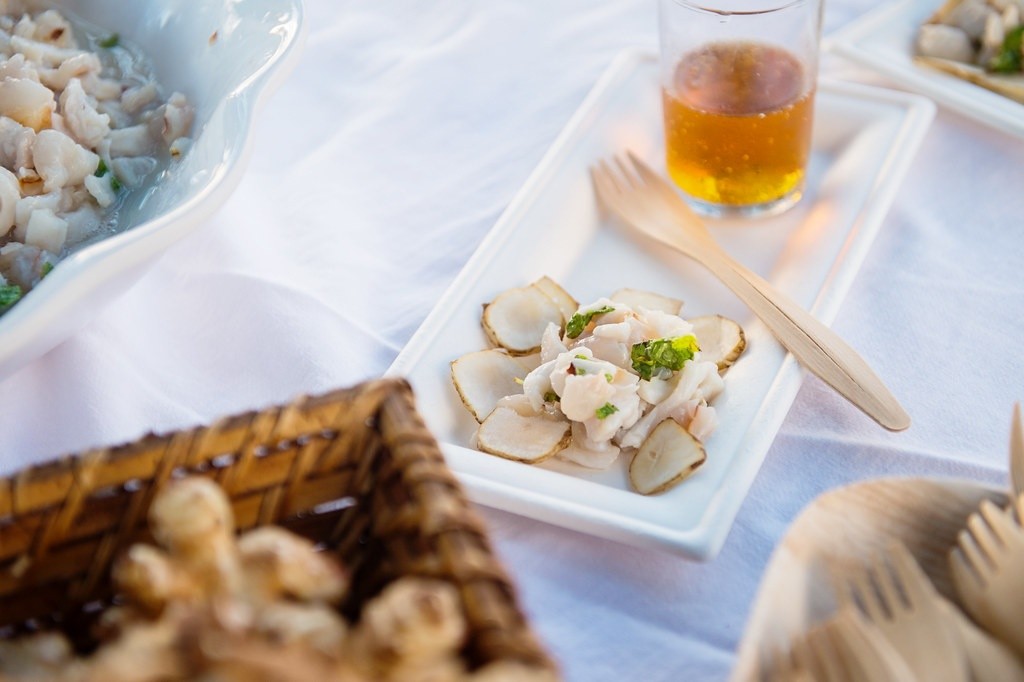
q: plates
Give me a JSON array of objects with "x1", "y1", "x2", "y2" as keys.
[
  {"x1": 379, "y1": 45, "x2": 939, "y2": 564},
  {"x1": 821, "y1": 0, "x2": 1024, "y2": 143}
]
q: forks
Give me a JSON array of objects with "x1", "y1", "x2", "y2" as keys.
[
  {"x1": 752, "y1": 404, "x2": 1024, "y2": 682},
  {"x1": 588, "y1": 149, "x2": 911, "y2": 432}
]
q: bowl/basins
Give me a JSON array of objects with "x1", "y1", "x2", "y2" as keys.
[{"x1": 0, "y1": 0, "x2": 302, "y2": 383}]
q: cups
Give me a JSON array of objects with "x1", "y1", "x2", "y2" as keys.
[{"x1": 657, "y1": 0, "x2": 826, "y2": 218}]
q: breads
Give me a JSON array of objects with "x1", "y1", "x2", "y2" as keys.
[{"x1": 0, "y1": 474, "x2": 539, "y2": 682}]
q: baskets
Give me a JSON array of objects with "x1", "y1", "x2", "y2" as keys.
[{"x1": 0, "y1": 379, "x2": 560, "y2": 682}]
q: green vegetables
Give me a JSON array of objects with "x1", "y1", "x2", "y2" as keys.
[
  {"x1": 543, "y1": 305, "x2": 704, "y2": 421},
  {"x1": 986, "y1": 22, "x2": 1024, "y2": 77}
]
